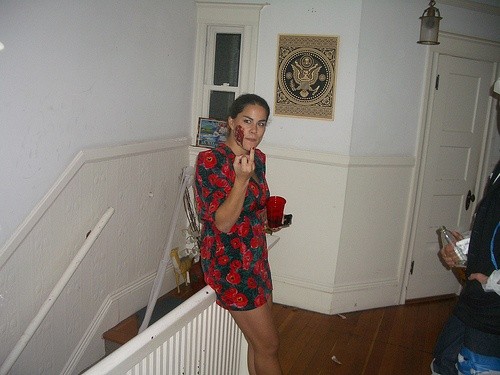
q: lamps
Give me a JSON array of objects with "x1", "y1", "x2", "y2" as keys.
[{"x1": 416, "y1": 0, "x2": 443, "y2": 46}]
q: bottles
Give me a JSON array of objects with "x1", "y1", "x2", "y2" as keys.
[{"x1": 438, "y1": 226, "x2": 468, "y2": 284}]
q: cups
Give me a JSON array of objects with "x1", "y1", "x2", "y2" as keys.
[{"x1": 265, "y1": 196, "x2": 286, "y2": 228}]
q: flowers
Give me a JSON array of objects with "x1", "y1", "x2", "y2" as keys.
[{"x1": 181, "y1": 186, "x2": 203, "y2": 263}]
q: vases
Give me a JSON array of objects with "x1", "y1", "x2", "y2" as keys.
[{"x1": 187, "y1": 256, "x2": 207, "y2": 293}]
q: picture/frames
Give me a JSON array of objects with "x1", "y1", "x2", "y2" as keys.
[{"x1": 196, "y1": 117, "x2": 230, "y2": 149}]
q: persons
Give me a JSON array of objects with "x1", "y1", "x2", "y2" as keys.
[
  {"x1": 431, "y1": 76, "x2": 500, "y2": 375},
  {"x1": 194, "y1": 94, "x2": 283, "y2": 375}
]
